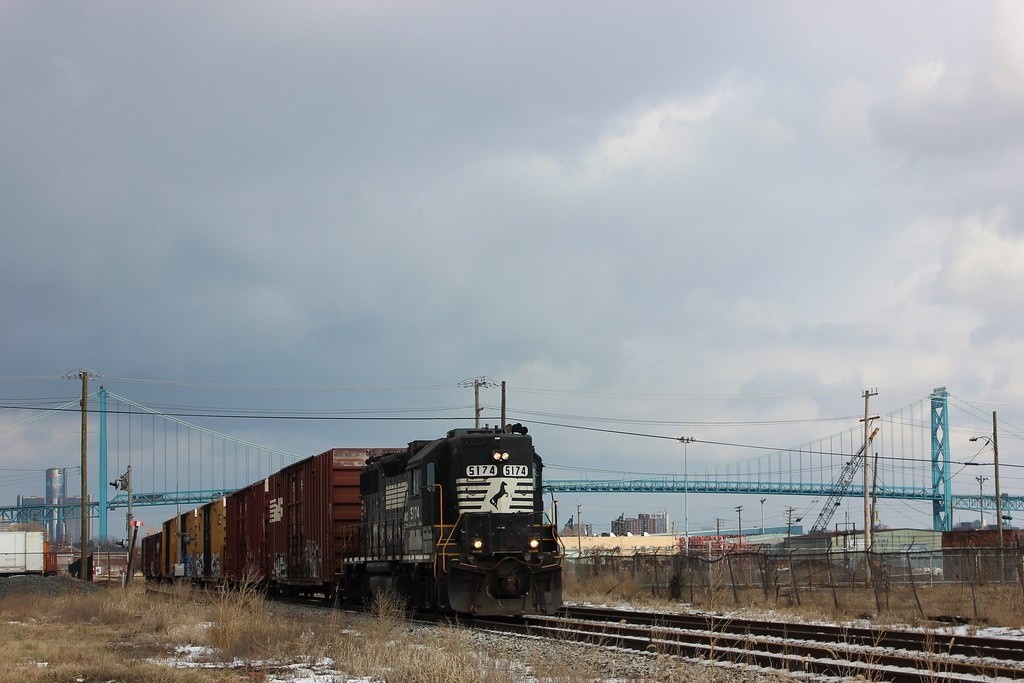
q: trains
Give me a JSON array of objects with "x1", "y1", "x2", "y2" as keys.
[{"x1": 139, "y1": 420, "x2": 566, "y2": 619}]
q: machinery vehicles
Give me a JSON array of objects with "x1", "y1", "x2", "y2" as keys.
[{"x1": 808, "y1": 427, "x2": 880, "y2": 534}]
